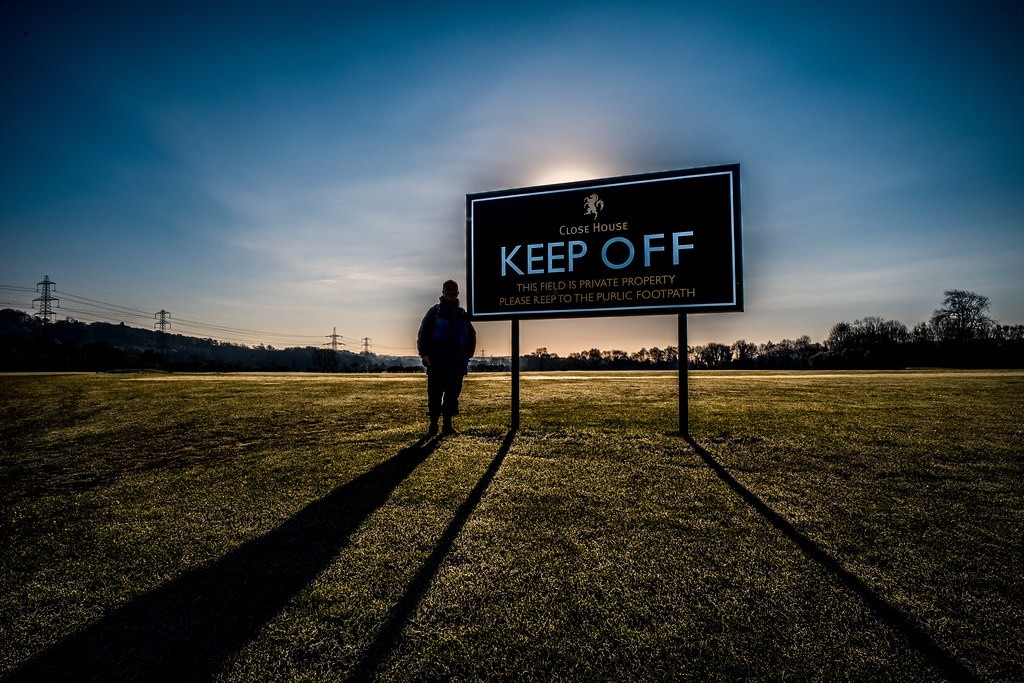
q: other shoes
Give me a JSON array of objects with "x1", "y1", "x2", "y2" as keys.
[
  {"x1": 428, "y1": 423, "x2": 439, "y2": 433},
  {"x1": 442, "y1": 424, "x2": 458, "y2": 434}
]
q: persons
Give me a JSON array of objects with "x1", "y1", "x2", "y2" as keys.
[{"x1": 417, "y1": 279, "x2": 476, "y2": 433}]
